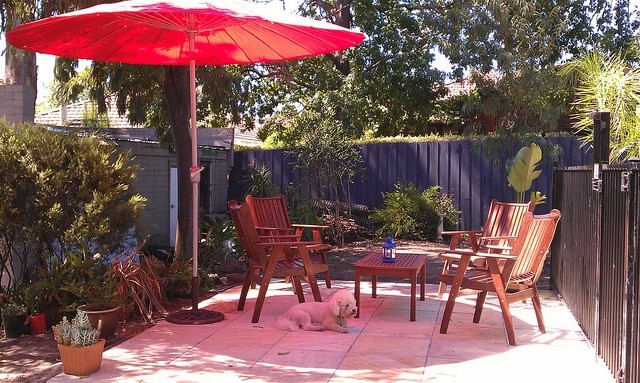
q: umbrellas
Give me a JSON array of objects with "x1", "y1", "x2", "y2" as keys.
[{"x1": 3, "y1": 0, "x2": 366, "y2": 325}]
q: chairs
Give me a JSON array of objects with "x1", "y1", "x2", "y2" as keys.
[
  {"x1": 437, "y1": 197, "x2": 533, "y2": 299},
  {"x1": 227, "y1": 199, "x2": 324, "y2": 323},
  {"x1": 439, "y1": 208, "x2": 561, "y2": 346},
  {"x1": 247, "y1": 192, "x2": 334, "y2": 289}
]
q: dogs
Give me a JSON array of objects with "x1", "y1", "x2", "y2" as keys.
[{"x1": 277, "y1": 289, "x2": 358, "y2": 334}]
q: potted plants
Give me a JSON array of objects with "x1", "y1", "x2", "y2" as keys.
[
  {"x1": 62, "y1": 251, "x2": 126, "y2": 340},
  {"x1": 52, "y1": 309, "x2": 105, "y2": 376},
  {"x1": 19, "y1": 277, "x2": 51, "y2": 336}
]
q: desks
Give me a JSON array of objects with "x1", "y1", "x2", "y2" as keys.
[{"x1": 353, "y1": 251, "x2": 427, "y2": 322}]
